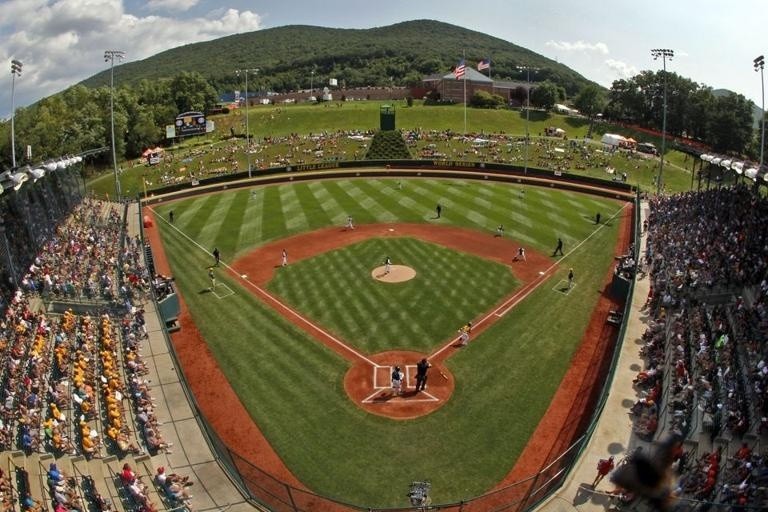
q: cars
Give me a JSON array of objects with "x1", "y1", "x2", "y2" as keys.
[{"x1": 260, "y1": 88, "x2": 318, "y2": 106}]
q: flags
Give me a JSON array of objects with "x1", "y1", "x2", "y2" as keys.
[
  {"x1": 477, "y1": 57, "x2": 489, "y2": 71},
  {"x1": 453, "y1": 55, "x2": 465, "y2": 81}
]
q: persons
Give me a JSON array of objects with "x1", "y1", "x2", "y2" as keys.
[
  {"x1": 212, "y1": 248, "x2": 220, "y2": 265},
  {"x1": 281, "y1": 248, "x2": 288, "y2": 266},
  {"x1": 589, "y1": 455, "x2": 614, "y2": 490},
  {"x1": 552, "y1": 238, "x2": 563, "y2": 256},
  {"x1": 403, "y1": 125, "x2": 670, "y2": 193},
  {"x1": 435, "y1": 203, "x2": 441, "y2": 217},
  {"x1": 513, "y1": 247, "x2": 524, "y2": 262},
  {"x1": 567, "y1": 267, "x2": 574, "y2": 288},
  {"x1": 389, "y1": 365, "x2": 405, "y2": 396},
  {"x1": 383, "y1": 255, "x2": 392, "y2": 275},
  {"x1": 518, "y1": 248, "x2": 526, "y2": 262},
  {"x1": 344, "y1": 215, "x2": 354, "y2": 230},
  {"x1": 496, "y1": 223, "x2": 504, "y2": 237},
  {"x1": 117, "y1": 123, "x2": 376, "y2": 188},
  {"x1": 0, "y1": 187, "x2": 195, "y2": 510},
  {"x1": 595, "y1": 213, "x2": 600, "y2": 224},
  {"x1": 605, "y1": 179, "x2": 768, "y2": 512},
  {"x1": 207, "y1": 268, "x2": 216, "y2": 292},
  {"x1": 412, "y1": 357, "x2": 432, "y2": 392},
  {"x1": 456, "y1": 321, "x2": 473, "y2": 346}
]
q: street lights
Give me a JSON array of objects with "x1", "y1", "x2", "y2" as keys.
[
  {"x1": 234, "y1": 64, "x2": 260, "y2": 180},
  {"x1": 10, "y1": 60, "x2": 24, "y2": 168},
  {"x1": 752, "y1": 54, "x2": 766, "y2": 184},
  {"x1": 104, "y1": 48, "x2": 127, "y2": 202},
  {"x1": 512, "y1": 63, "x2": 541, "y2": 179},
  {"x1": 649, "y1": 49, "x2": 676, "y2": 197}
]
